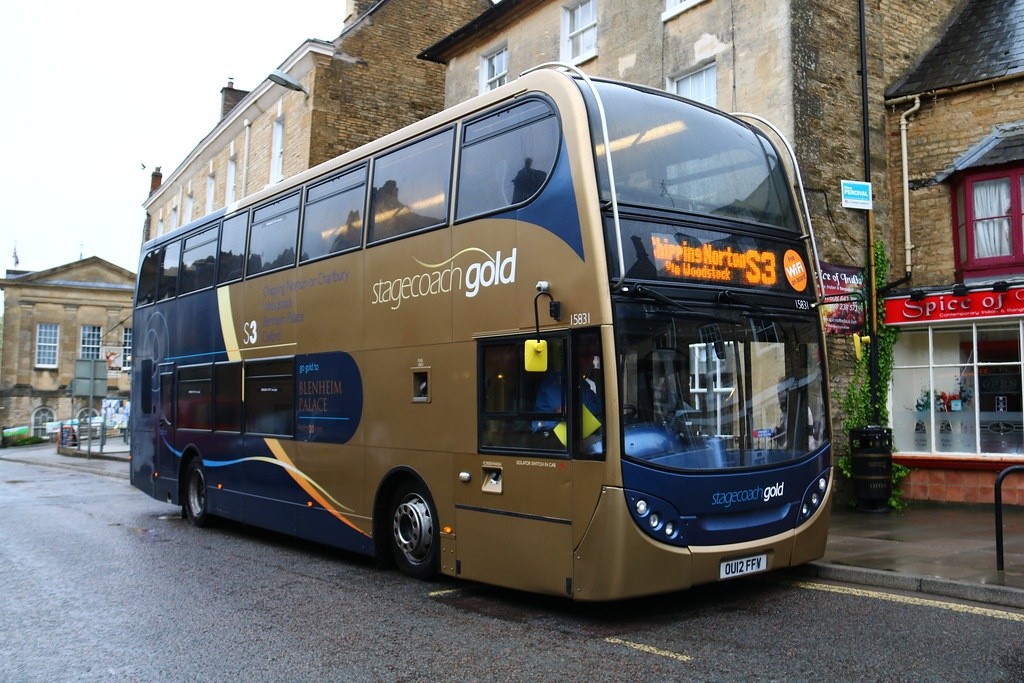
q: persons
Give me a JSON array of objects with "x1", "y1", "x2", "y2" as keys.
[{"x1": 530, "y1": 341, "x2": 603, "y2": 447}]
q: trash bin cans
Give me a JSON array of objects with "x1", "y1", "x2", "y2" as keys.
[{"x1": 849, "y1": 425, "x2": 894, "y2": 516}]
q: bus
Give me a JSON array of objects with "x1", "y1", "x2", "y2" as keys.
[{"x1": 128, "y1": 62, "x2": 835, "y2": 606}]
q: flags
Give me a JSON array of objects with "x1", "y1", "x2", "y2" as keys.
[{"x1": 813, "y1": 261, "x2": 866, "y2": 335}]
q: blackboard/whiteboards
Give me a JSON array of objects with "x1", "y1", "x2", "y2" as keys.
[{"x1": 56, "y1": 426, "x2": 78, "y2": 448}]
